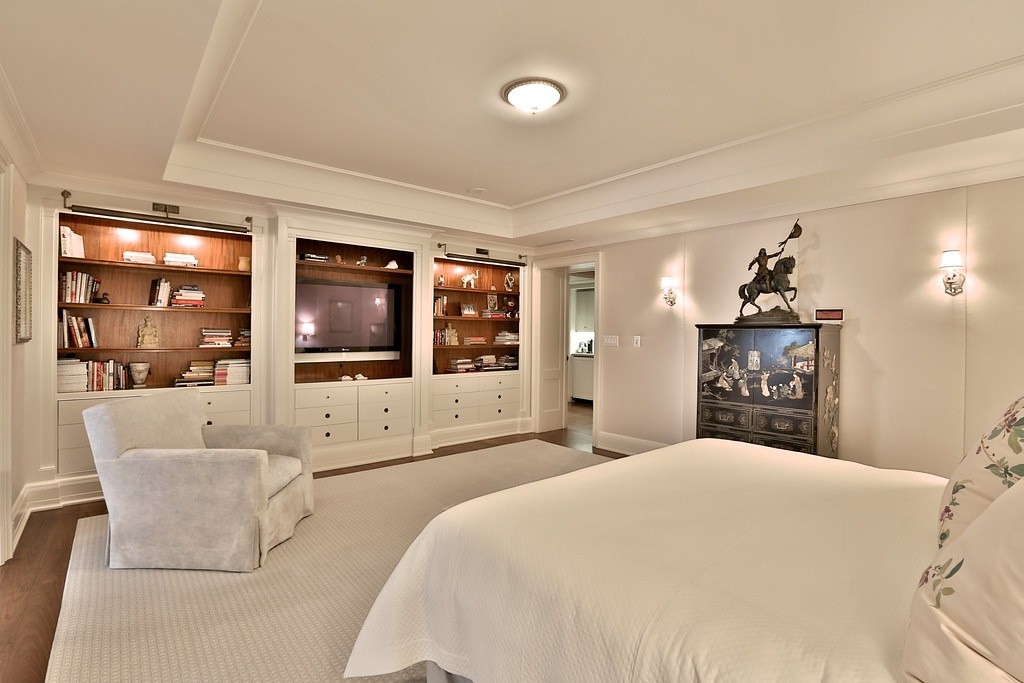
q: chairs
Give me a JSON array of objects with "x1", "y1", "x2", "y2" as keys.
[{"x1": 80, "y1": 388, "x2": 315, "y2": 574}]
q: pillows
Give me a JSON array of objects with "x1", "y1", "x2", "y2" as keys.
[
  {"x1": 938, "y1": 395, "x2": 1024, "y2": 550},
  {"x1": 901, "y1": 478, "x2": 1024, "y2": 683}
]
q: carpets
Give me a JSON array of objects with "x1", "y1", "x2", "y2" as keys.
[{"x1": 44, "y1": 439, "x2": 639, "y2": 683}]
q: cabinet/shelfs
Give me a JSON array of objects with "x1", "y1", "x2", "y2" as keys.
[
  {"x1": 572, "y1": 357, "x2": 594, "y2": 400},
  {"x1": 58, "y1": 213, "x2": 254, "y2": 354},
  {"x1": 433, "y1": 284, "x2": 520, "y2": 348},
  {"x1": 695, "y1": 323, "x2": 844, "y2": 460},
  {"x1": 57, "y1": 390, "x2": 251, "y2": 475},
  {"x1": 429, "y1": 375, "x2": 520, "y2": 431},
  {"x1": 295, "y1": 382, "x2": 413, "y2": 446}
]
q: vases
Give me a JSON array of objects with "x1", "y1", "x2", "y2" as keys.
[{"x1": 238, "y1": 256, "x2": 251, "y2": 272}]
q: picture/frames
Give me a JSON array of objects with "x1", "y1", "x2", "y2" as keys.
[{"x1": 14, "y1": 236, "x2": 32, "y2": 342}]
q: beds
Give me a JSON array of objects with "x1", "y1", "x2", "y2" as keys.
[{"x1": 342, "y1": 426, "x2": 950, "y2": 683}]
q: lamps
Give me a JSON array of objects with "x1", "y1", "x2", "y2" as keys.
[
  {"x1": 437, "y1": 242, "x2": 528, "y2": 268},
  {"x1": 659, "y1": 276, "x2": 677, "y2": 306},
  {"x1": 60, "y1": 189, "x2": 254, "y2": 234},
  {"x1": 939, "y1": 250, "x2": 966, "y2": 296},
  {"x1": 500, "y1": 77, "x2": 567, "y2": 115}
]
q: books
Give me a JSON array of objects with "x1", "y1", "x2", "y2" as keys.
[
  {"x1": 60, "y1": 226, "x2": 85, "y2": 258},
  {"x1": 199, "y1": 327, "x2": 251, "y2": 348},
  {"x1": 123, "y1": 251, "x2": 157, "y2": 264},
  {"x1": 434, "y1": 295, "x2": 448, "y2": 316},
  {"x1": 148, "y1": 278, "x2": 205, "y2": 308},
  {"x1": 56, "y1": 309, "x2": 100, "y2": 348},
  {"x1": 446, "y1": 355, "x2": 505, "y2": 372},
  {"x1": 174, "y1": 358, "x2": 251, "y2": 387},
  {"x1": 464, "y1": 335, "x2": 487, "y2": 345},
  {"x1": 492, "y1": 332, "x2": 519, "y2": 345},
  {"x1": 59, "y1": 271, "x2": 102, "y2": 303},
  {"x1": 57, "y1": 358, "x2": 130, "y2": 392},
  {"x1": 482, "y1": 309, "x2": 505, "y2": 318},
  {"x1": 163, "y1": 253, "x2": 198, "y2": 267},
  {"x1": 433, "y1": 329, "x2": 447, "y2": 345}
]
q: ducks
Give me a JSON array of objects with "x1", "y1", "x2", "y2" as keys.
[{"x1": 91, "y1": 291, "x2": 111, "y2": 305}]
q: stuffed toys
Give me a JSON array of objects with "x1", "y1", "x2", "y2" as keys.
[{"x1": 502, "y1": 297, "x2": 520, "y2": 318}]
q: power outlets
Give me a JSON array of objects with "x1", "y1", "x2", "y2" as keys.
[{"x1": 634, "y1": 336, "x2": 640, "y2": 347}]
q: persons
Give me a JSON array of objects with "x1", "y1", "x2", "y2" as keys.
[{"x1": 138, "y1": 317, "x2": 159, "y2": 344}]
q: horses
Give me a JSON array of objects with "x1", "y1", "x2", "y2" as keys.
[{"x1": 737, "y1": 255, "x2": 799, "y2": 318}]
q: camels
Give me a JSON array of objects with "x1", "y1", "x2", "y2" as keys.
[{"x1": 460, "y1": 269, "x2": 480, "y2": 290}]
